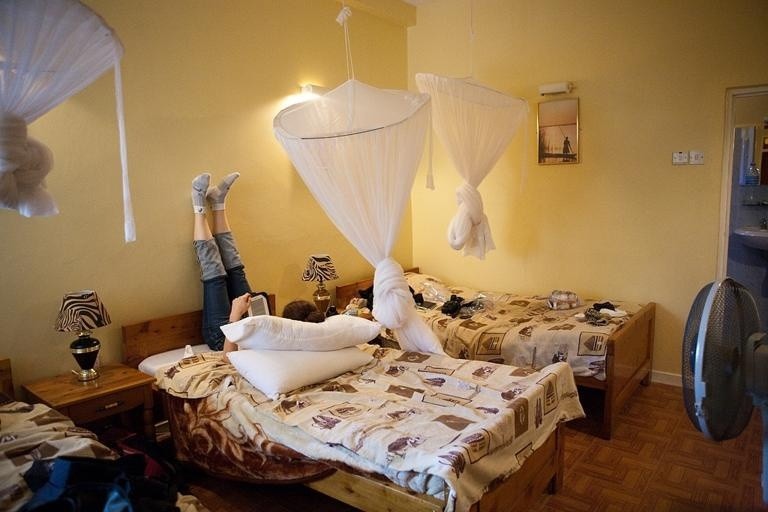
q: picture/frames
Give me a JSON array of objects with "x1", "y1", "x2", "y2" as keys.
[{"x1": 536, "y1": 95, "x2": 580, "y2": 165}]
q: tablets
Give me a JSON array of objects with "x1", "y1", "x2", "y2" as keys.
[{"x1": 248, "y1": 294, "x2": 270, "y2": 317}]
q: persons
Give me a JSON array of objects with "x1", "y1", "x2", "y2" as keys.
[
  {"x1": 190, "y1": 172, "x2": 326, "y2": 365},
  {"x1": 562, "y1": 157, "x2": 571, "y2": 162},
  {"x1": 562, "y1": 137, "x2": 571, "y2": 155}
]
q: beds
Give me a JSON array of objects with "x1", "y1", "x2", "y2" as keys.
[
  {"x1": 0, "y1": 359, "x2": 210, "y2": 512},
  {"x1": 121, "y1": 293, "x2": 587, "y2": 512},
  {"x1": 335, "y1": 269, "x2": 656, "y2": 440}
]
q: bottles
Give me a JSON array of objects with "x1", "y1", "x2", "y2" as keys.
[{"x1": 744, "y1": 162, "x2": 760, "y2": 205}]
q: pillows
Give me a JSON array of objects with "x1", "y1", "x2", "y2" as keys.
[
  {"x1": 220, "y1": 314, "x2": 382, "y2": 351},
  {"x1": 226, "y1": 347, "x2": 375, "y2": 401}
]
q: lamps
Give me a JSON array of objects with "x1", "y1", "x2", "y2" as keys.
[
  {"x1": 53, "y1": 289, "x2": 112, "y2": 382},
  {"x1": 301, "y1": 255, "x2": 340, "y2": 314}
]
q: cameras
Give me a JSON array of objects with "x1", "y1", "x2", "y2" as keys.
[{"x1": 442, "y1": 301, "x2": 458, "y2": 314}]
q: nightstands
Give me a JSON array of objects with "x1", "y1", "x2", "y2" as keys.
[{"x1": 20, "y1": 362, "x2": 157, "y2": 445}]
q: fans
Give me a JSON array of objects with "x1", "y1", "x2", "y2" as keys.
[{"x1": 680, "y1": 277, "x2": 768, "y2": 505}]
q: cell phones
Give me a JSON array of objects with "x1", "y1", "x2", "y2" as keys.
[{"x1": 418, "y1": 300, "x2": 436, "y2": 310}]
